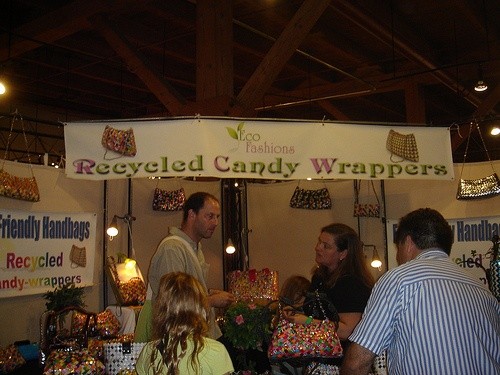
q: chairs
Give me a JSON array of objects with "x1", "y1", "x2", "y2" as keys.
[{"x1": 36, "y1": 304, "x2": 106, "y2": 372}]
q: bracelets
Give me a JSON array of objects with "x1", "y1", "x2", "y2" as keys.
[{"x1": 306, "y1": 316, "x2": 312, "y2": 324}]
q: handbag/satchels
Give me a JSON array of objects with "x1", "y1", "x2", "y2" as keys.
[
  {"x1": 0, "y1": 255, "x2": 147, "y2": 375},
  {"x1": 290, "y1": 186, "x2": 332, "y2": 210},
  {"x1": 226, "y1": 269, "x2": 280, "y2": 311},
  {"x1": 305, "y1": 362, "x2": 339, "y2": 375},
  {"x1": 69, "y1": 245, "x2": 86, "y2": 269},
  {"x1": 354, "y1": 203, "x2": 381, "y2": 218},
  {"x1": 0, "y1": 169, "x2": 40, "y2": 202},
  {"x1": 153, "y1": 187, "x2": 186, "y2": 212},
  {"x1": 456, "y1": 174, "x2": 500, "y2": 200},
  {"x1": 384, "y1": 130, "x2": 420, "y2": 162},
  {"x1": 267, "y1": 290, "x2": 343, "y2": 358},
  {"x1": 101, "y1": 125, "x2": 136, "y2": 160}
]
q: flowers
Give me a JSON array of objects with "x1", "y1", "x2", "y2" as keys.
[{"x1": 226, "y1": 297, "x2": 273, "y2": 350}]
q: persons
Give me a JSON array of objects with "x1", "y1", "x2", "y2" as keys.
[
  {"x1": 281, "y1": 223, "x2": 374, "y2": 375},
  {"x1": 340, "y1": 207, "x2": 500, "y2": 375},
  {"x1": 136, "y1": 272, "x2": 234, "y2": 375},
  {"x1": 270, "y1": 274, "x2": 311, "y2": 375},
  {"x1": 148, "y1": 192, "x2": 233, "y2": 339}
]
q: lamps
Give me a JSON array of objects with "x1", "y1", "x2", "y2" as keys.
[
  {"x1": 361, "y1": 242, "x2": 383, "y2": 268},
  {"x1": 472, "y1": 62, "x2": 489, "y2": 92},
  {"x1": 225, "y1": 237, "x2": 248, "y2": 271},
  {"x1": 108, "y1": 214, "x2": 137, "y2": 259}
]
